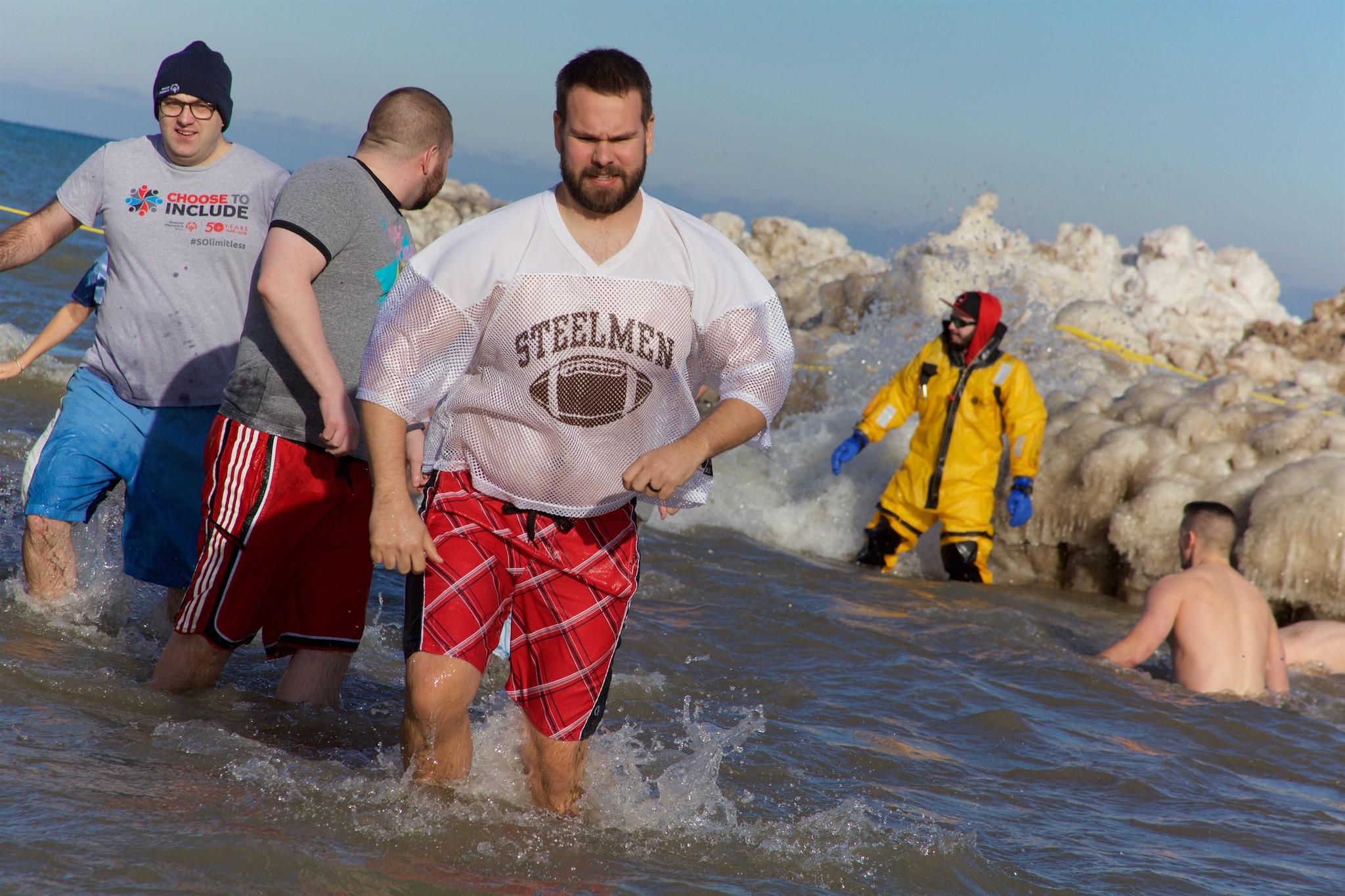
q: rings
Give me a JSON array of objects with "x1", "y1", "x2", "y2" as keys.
[{"x1": 648, "y1": 483, "x2": 661, "y2": 492}]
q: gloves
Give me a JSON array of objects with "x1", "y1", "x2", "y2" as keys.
[
  {"x1": 832, "y1": 430, "x2": 869, "y2": 476},
  {"x1": 1008, "y1": 475, "x2": 1033, "y2": 526}
]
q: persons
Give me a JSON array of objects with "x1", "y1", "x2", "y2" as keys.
[
  {"x1": 152, "y1": 85, "x2": 455, "y2": 696},
  {"x1": 1098, "y1": 501, "x2": 1290, "y2": 697},
  {"x1": 831, "y1": 291, "x2": 1048, "y2": 581},
  {"x1": 355, "y1": 47, "x2": 791, "y2": 811},
  {"x1": 1277, "y1": 620, "x2": 1345, "y2": 678},
  {"x1": 0, "y1": 40, "x2": 294, "y2": 623}
]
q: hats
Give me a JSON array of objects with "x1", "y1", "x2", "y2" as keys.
[
  {"x1": 153, "y1": 40, "x2": 234, "y2": 133},
  {"x1": 942, "y1": 290, "x2": 980, "y2": 321}
]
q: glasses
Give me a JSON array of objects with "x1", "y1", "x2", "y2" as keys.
[
  {"x1": 950, "y1": 314, "x2": 977, "y2": 328},
  {"x1": 160, "y1": 98, "x2": 217, "y2": 119}
]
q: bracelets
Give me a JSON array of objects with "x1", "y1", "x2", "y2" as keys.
[{"x1": 13, "y1": 357, "x2": 24, "y2": 373}]
